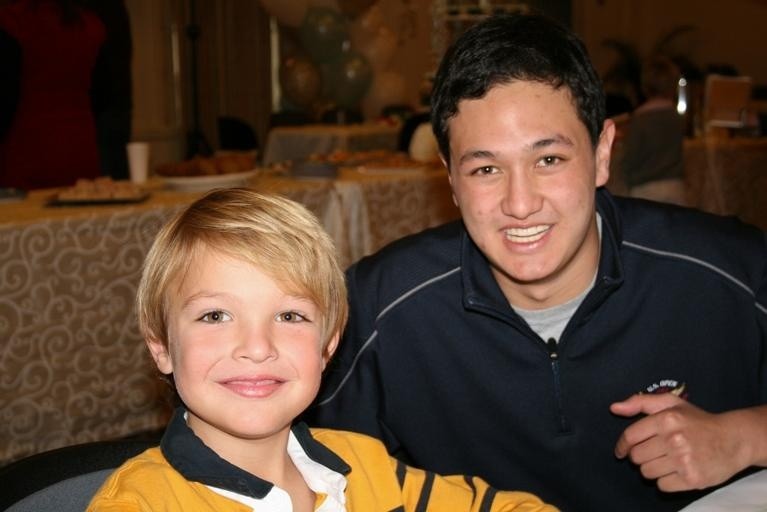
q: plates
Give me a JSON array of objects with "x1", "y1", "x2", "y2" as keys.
[{"x1": 161, "y1": 167, "x2": 262, "y2": 190}]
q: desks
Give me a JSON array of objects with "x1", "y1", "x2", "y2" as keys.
[{"x1": 0, "y1": 148, "x2": 452, "y2": 463}]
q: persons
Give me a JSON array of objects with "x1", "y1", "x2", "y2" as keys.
[
  {"x1": 293, "y1": 13, "x2": 767, "y2": 512},
  {"x1": 87, "y1": 187, "x2": 565, "y2": 512}
]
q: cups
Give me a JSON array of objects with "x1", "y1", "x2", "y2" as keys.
[{"x1": 126, "y1": 142, "x2": 150, "y2": 184}]
